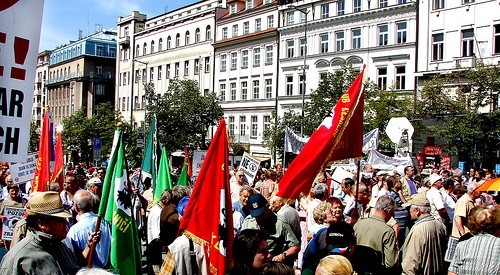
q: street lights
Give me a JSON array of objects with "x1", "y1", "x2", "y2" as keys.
[
  {"x1": 287, "y1": 5, "x2": 311, "y2": 137},
  {"x1": 133, "y1": 59, "x2": 151, "y2": 105}
]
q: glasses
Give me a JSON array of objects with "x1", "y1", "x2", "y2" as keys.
[
  {"x1": 44, "y1": 216, "x2": 67, "y2": 223},
  {"x1": 98, "y1": 172, "x2": 104, "y2": 174},
  {"x1": 239, "y1": 175, "x2": 245, "y2": 178},
  {"x1": 414, "y1": 181, "x2": 420, "y2": 185}
]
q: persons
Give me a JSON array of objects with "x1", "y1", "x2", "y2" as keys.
[{"x1": 0, "y1": 159, "x2": 500, "y2": 275}]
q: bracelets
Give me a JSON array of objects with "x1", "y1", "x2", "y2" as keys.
[{"x1": 282, "y1": 253, "x2": 287, "y2": 258}]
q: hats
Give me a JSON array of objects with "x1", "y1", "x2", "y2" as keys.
[
  {"x1": 88, "y1": 168, "x2": 94, "y2": 172},
  {"x1": 421, "y1": 169, "x2": 430, "y2": 175},
  {"x1": 429, "y1": 173, "x2": 442, "y2": 186},
  {"x1": 377, "y1": 171, "x2": 389, "y2": 176},
  {"x1": 68, "y1": 170, "x2": 73, "y2": 174},
  {"x1": 26, "y1": 190, "x2": 72, "y2": 218},
  {"x1": 402, "y1": 195, "x2": 431, "y2": 208},
  {"x1": 175, "y1": 195, "x2": 190, "y2": 216},
  {"x1": 453, "y1": 168, "x2": 463, "y2": 178},
  {"x1": 88, "y1": 177, "x2": 102, "y2": 184},
  {"x1": 326, "y1": 222, "x2": 355, "y2": 254},
  {"x1": 247, "y1": 193, "x2": 269, "y2": 217}
]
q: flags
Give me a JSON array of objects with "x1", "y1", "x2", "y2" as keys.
[
  {"x1": 32, "y1": 111, "x2": 65, "y2": 194},
  {"x1": 141, "y1": 115, "x2": 163, "y2": 201},
  {"x1": 153, "y1": 147, "x2": 172, "y2": 203},
  {"x1": 180, "y1": 117, "x2": 235, "y2": 275},
  {"x1": 276, "y1": 71, "x2": 362, "y2": 199},
  {"x1": 98, "y1": 129, "x2": 143, "y2": 275},
  {"x1": 177, "y1": 148, "x2": 192, "y2": 188}
]
